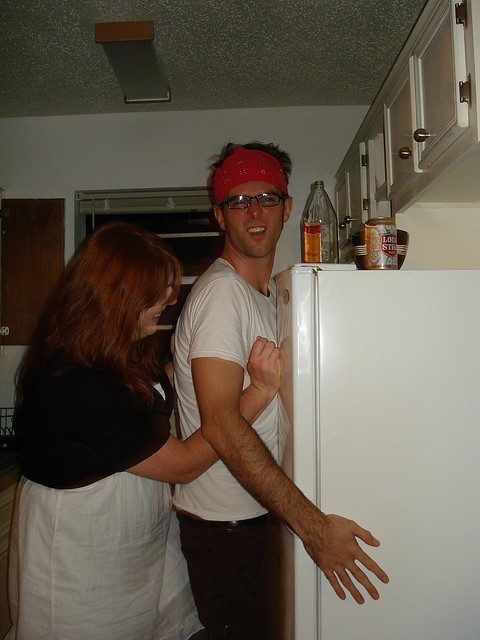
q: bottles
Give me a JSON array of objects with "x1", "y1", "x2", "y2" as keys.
[{"x1": 300, "y1": 180, "x2": 338, "y2": 263}]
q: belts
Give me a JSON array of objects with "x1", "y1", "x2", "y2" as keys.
[{"x1": 177, "y1": 508, "x2": 274, "y2": 527}]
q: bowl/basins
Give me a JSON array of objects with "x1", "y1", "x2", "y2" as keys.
[{"x1": 352, "y1": 230, "x2": 409, "y2": 270}]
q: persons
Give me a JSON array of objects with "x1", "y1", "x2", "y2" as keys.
[
  {"x1": 2, "y1": 221, "x2": 285, "y2": 640},
  {"x1": 172, "y1": 143, "x2": 389, "y2": 640}
]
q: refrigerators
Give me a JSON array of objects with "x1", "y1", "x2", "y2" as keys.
[{"x1": 276, "y1": 262, "x2": 479, "y2": 640}]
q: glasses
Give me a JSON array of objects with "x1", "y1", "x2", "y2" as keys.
[{"x1": 217, "y1": 191, "x2": 286, "y2": 209}]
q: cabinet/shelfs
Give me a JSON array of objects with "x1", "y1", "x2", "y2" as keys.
[
  {"x1": 336, "y1": 95, "x2": 390, "y2": 256},
  {"x1": 384, "y1": 0, "x2": 480, "y2": 213},
  {"x1": 1, "y1": 198, "x2": 65, "y2": 345}
]
